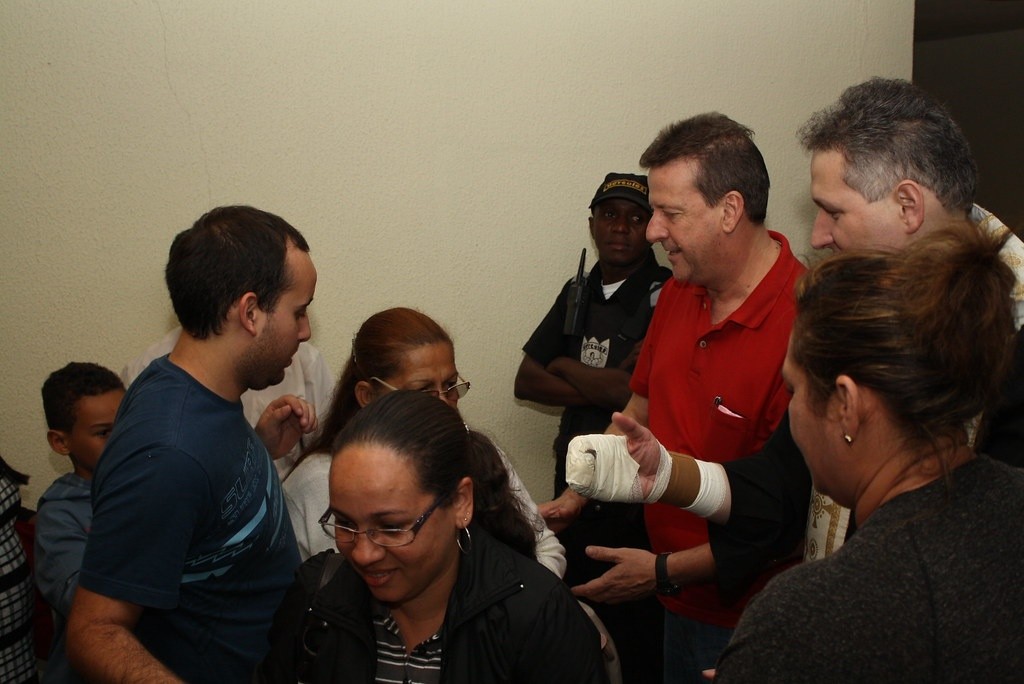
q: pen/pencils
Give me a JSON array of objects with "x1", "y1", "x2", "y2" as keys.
[{"x1": 715, "y1": 395, "x2": 723, "y2": 405}]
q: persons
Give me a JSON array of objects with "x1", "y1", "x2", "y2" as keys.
[
  {"x1": 515, "y1": 172, "x2": 665, "y2": 684},
  {"x1": 537, "y1": 111, "x2": 811, "y2": 684},
  {"x1": 564, "y1": 80, "x2": 1024, "y2": 684},
  {"x1": 67, "y1": 204, "x2": 338, "y2": 684},
  {"x1": 713, "y1": 219, "x2": 1024, "y2": 684},
  {"x1": 31, "y1": 362, "x2": 124, "y2": 684},
  {"x1": 256, "y1": 389, "x2": 615, "y2": 684},
  {"x1": 0, "y1": 456, "x2": 44, "y2": 684},
  {"x1": 279, "y1": 307, "x2": 622, "y2": 684}
]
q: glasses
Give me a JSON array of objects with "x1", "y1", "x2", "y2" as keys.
[{"x1": 367, "y1": 372, "x2": 471, "y2": 400}]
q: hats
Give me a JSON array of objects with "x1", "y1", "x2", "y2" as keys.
[{"x1": 588, "y1": 172, "x2": 654, "y2": 217}]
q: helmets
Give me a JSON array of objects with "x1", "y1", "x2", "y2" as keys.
[{"x1": 317, "y1": 488, "x2": 449, "y2": 547}]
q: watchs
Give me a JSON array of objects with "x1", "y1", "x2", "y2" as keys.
[{"x1": 655, "y1": 552, "x2": 684, "y2": 595}]
